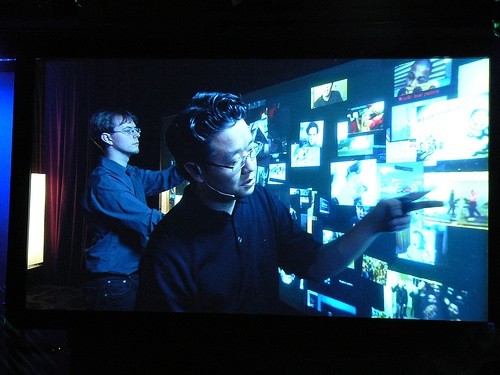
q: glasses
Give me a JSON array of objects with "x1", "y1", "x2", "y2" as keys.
[
  {"x1": 200, "y1": 140, "x2": 264, "y2": 172},
  {"x1": 115, "y1": 127, "x2": 143, "y2": 136}
]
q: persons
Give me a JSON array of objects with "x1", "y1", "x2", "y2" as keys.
[
  {"x1": 301, "y1": 122, "x2": 321, "y2": 147},
  {"x1": 362, "y1": 256, "x2": 387, "y2": 283},
  {"x1": 447, "y1": 190, "x2": 456, "y2": 215},
  {"x1": 398, "y1": 60, "x2": 432, "y2": 96},
  {"x1": 137, "y1": 93, "x2": 442, "y2": 314},
  {"x1": 411, "y1": 106, "x2": 455, "y2": 147},
  {"x1": 391, "y1": 279, "x2": 465, "y2": 321},
  {"x1": 360, "y1": 112, "x2": 373, "y2": 132},
  {"x1": 82, "y1": 111, "x2": 185, "y2": 312},
  {"x1": 398, "y1": 231, "x2": 430, "y2": 262},
  {"x1": 313, "y1": 82, "x2": 343, "y2": 109},
  {"x1": 468, "y1": 191, "x2": 477, "y2": 218},
  {"x1": 353, "y1": 182, "x2": 367, "y2": 205}
]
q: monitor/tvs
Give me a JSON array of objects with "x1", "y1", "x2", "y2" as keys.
[
  {"x1": 0, "y1": 53, "x2": 20, "y2": 316},
  {"x1": 158, "y1": 57, "x2": 490, "y2": 324}
]
